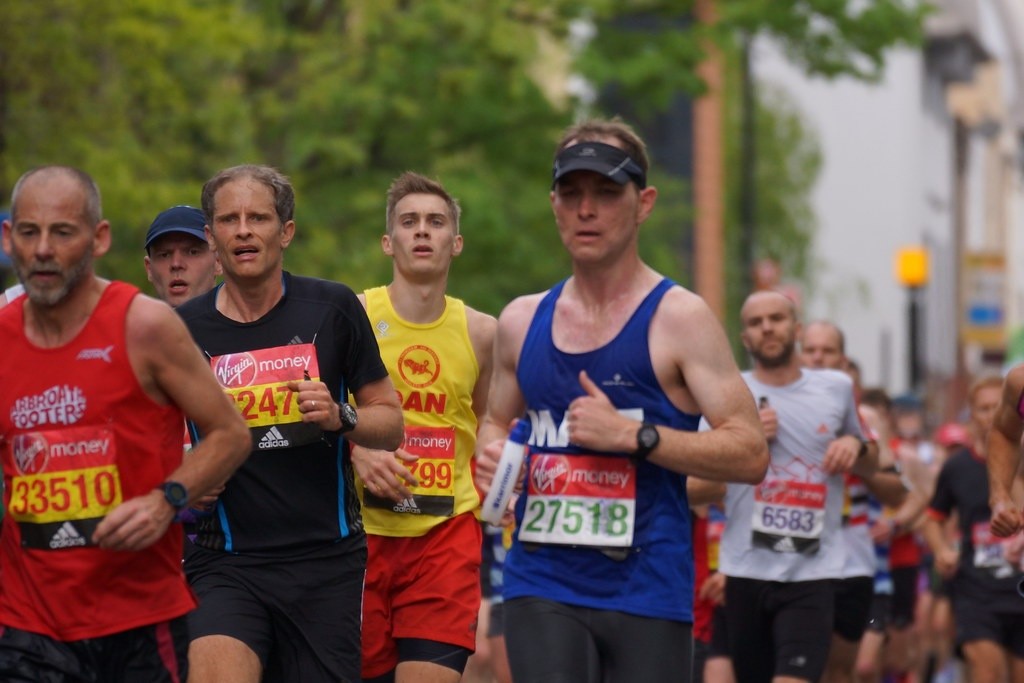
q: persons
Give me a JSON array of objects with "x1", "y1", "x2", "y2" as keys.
[
  {"x1": 475, "y1": 122, "x2": 771, "y2": 683},
  {"x1": 685, "y1": 293, "x2": 1024, "y2": 683},
  {"x1": 0, "y1": 165, "x2": 532, "y2": 683}
]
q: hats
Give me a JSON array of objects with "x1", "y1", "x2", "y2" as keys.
[
  {"x1": 934, "y1": 423, "x2": 971, "y2": 449},
  {"x1": 144, "y1": 206, "x2": 208, "y2": 258},
  {"x1": 551, "y1": 141, "x2": 646, "y2": 191}
]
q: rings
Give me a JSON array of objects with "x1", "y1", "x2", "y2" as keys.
[{"x1": 312, "y1": 401, "x2": 317, "y2": 409}]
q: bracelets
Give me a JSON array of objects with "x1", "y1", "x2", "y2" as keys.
[{"x1": 855, "y1": 435, "x2": 868, "y2": 457}]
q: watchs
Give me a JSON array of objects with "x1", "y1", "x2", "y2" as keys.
[
  {"x1": 157, "y1": 481, "x2": 187, "y2": 521},
  {"x1": 332, "y1": 402, "x2": 358, "y2": 434},
  {"x1": 632, "y1": 423, "x2": 659, "y2": 461}
]
